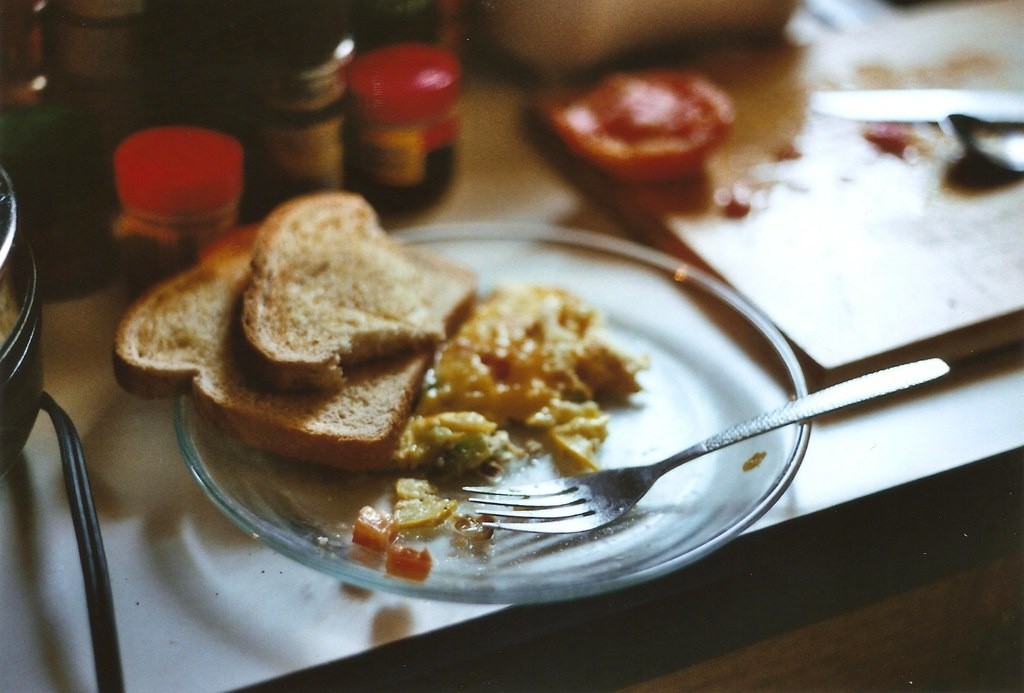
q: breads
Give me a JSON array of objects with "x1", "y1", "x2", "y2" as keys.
[
  {"x1": 238, "y1": 192, "x2": 448, "y2": 394},
  {"x1": 110, "y1": 220, "x2": 476, "y2": 472}
]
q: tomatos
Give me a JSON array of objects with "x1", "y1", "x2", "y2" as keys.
[{"x1": 554, "y1": 71, "x2": 740, "y2": 181}]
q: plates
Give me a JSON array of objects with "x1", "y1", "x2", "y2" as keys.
[{"x1": 174, "y1": 221, "x2": 812, "y2": 606}]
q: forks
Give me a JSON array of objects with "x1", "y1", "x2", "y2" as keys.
[{"x1": 462, "y1": 357, "x2": 950, "y2": 533}]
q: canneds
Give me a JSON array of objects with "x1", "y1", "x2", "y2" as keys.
[
  {"x1": 250, "y1": 39, "x2": 462, "y2": 215},
  {"x1": 113, "y1": 127, "x2": 243, "y2": 320}
]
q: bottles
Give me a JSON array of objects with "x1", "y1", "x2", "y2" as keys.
[
  {"x1": 350, "y1": 46, "x2": 460, "y2": 205},
  {"x1": 242, "y1": 30, "x2": 358, "y2": 197},
  {"x1": 42, "y1": 0, "x2": 146, "y2": 84},
  {"x1": 111, "y1": 125, "x2": 244, "y2": 308}
]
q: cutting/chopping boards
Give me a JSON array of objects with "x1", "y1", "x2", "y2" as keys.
[{"x1": 520, "y1": 0, "x2": 1024, "y2": 388}]
